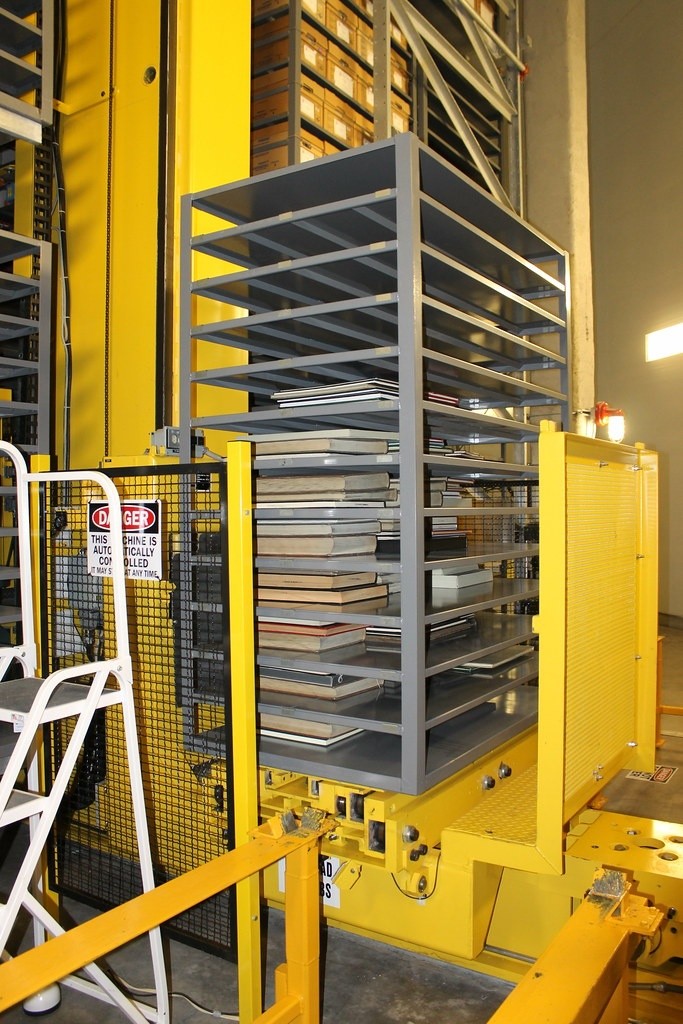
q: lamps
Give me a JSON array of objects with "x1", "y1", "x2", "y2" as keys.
[{"x1": 578, "y1": 401, "x2": 626, "y2": 445}]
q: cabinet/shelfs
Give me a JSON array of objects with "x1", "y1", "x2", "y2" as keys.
[
  {"x1": 0, "y1": 228, "x2": 53, "y2": 776},
  {"x1": 251, "y1": 0, "x2": 416, "y2": 165},
  {"x1": 417, "y1": 35, "x2": 505, "y2": 198},
  {"x1": 0, "y1": 0, "x2": 54, "y2": 144},
  {"x1": 179, "y1": 132, "x2": 576, "y2": 798}
]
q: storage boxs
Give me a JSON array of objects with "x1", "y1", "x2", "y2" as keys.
[
  {"x1": 250, "y1": 0, "x2": 411, "y2": 177},
  {"x1": 463, "y1": 0, "x2": 498, "y2": 30}
]
